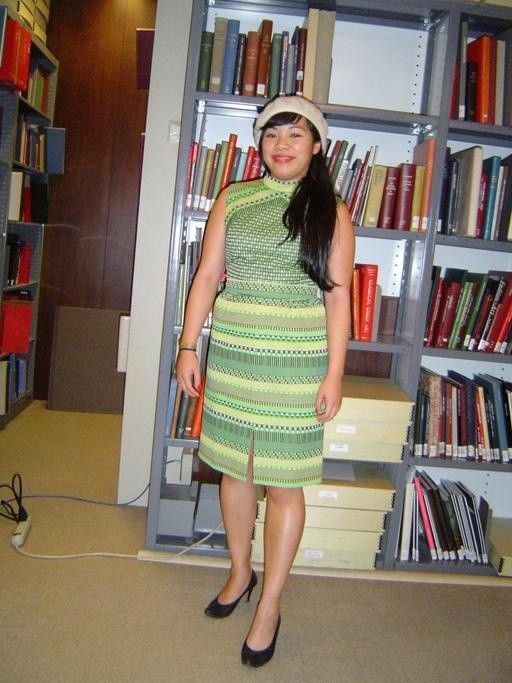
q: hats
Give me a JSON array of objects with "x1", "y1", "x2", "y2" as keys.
[{"x1": 253, "y1": 97, "x2": 328, "y2": 157}]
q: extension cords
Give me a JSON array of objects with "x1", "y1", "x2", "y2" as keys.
[{"x1": 11, "y1": 516, "x2": 32, "y2": 546}]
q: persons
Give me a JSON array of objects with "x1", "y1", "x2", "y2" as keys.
[{"x1": 175, "y1": 97, "x2": 355, "y2": 668}]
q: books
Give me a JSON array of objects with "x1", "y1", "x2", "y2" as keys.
[
  {"x1": 0, "y1": 20, "x2": 67, "y2": 415},
  {"x1": 167, "y1": 8, "x2": 436, "y2": 484},
  {"x1": 396, "y1": 20, "x2": 512, "y2": 565}
]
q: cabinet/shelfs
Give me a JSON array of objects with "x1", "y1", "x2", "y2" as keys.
[
  {"x1": 0, "y1": 4, "x2": 65, "y2": 425},
  {"x1": 146, "y1": 0, "x2": 512, "y2": 583}
]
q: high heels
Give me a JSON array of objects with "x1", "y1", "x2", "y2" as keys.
[
  {"x1": 241, "y1": 615, "x2": 280, "y2": 667},
  {"x1": 204, "y1": 568, "x2": 257, "y2": 618}
]
